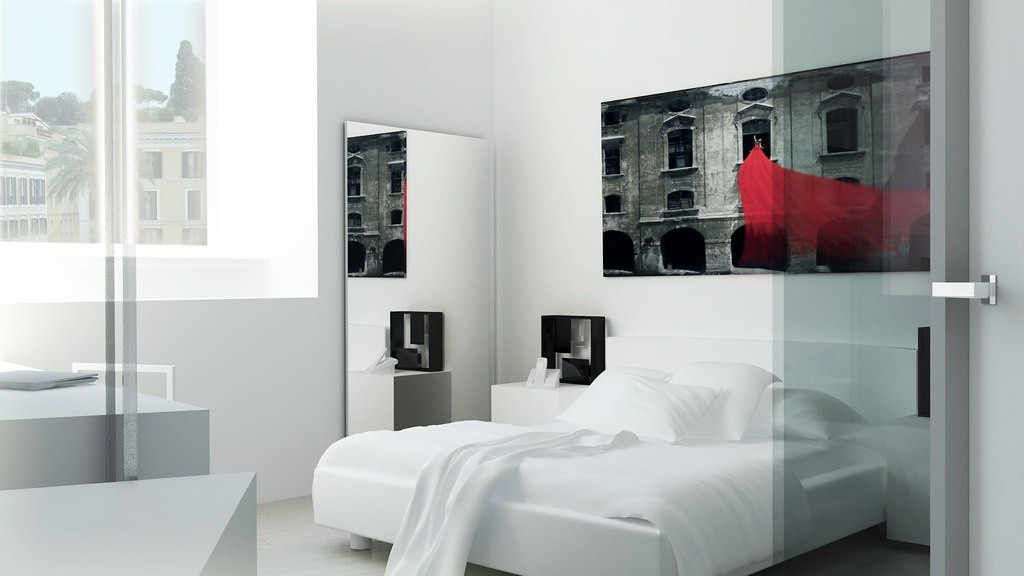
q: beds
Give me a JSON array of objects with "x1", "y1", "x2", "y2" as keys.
[{"x1": 310, "y1": 336, "x2": 918, "y2": 574}]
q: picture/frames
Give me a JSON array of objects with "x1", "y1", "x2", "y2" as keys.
[
  {"x1": 345, "y1": 130, "x2": 407, "y2": 278},
  {"x1": 601, "y1": 53, "x2": 931, "y2": 276}
]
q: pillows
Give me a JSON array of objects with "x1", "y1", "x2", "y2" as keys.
[
  {"x1": 560, "y1": 364, "x2": 722, "y2": 445},
  {"x1": 747, "y1": 387, "x2": 862, "y2": 442},
  {"x1": 621, "y1": 361, "x2": 782, "y2": 442}
]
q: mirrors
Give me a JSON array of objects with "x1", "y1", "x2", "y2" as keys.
[{"x1": 343, "y1": 119, "x2": 497, "y2": 434}]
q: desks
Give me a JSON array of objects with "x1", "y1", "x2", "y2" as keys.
[
  {"x1": 491, "y1": 382, "x2": 590, "y2": 427},
  {"x1": 345, "y1": 369, "x2": 452, "y2": 433}
]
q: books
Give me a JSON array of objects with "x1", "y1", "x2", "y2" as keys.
[{"x1": 0, "y1": 370, "x2": 98, "y2": 389}]
